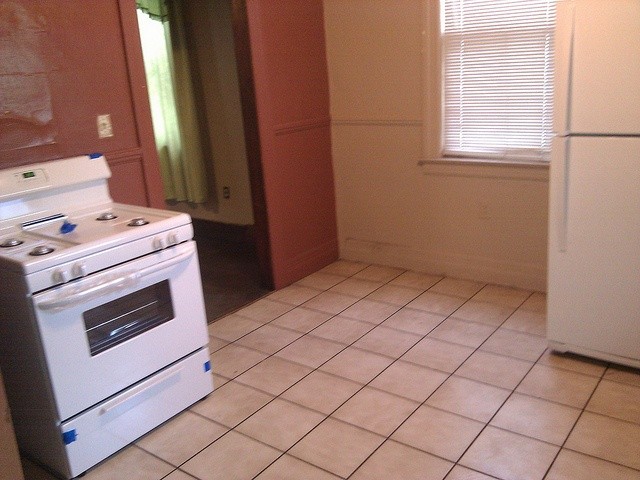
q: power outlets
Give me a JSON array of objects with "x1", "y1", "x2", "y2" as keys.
[{"x1": 96, "y1": 114, "x2": 114, "y2": 138}]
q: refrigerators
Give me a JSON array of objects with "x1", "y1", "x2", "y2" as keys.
[{"x1": 548, "y1": 1, "x2": 640, "y2": 369}]
